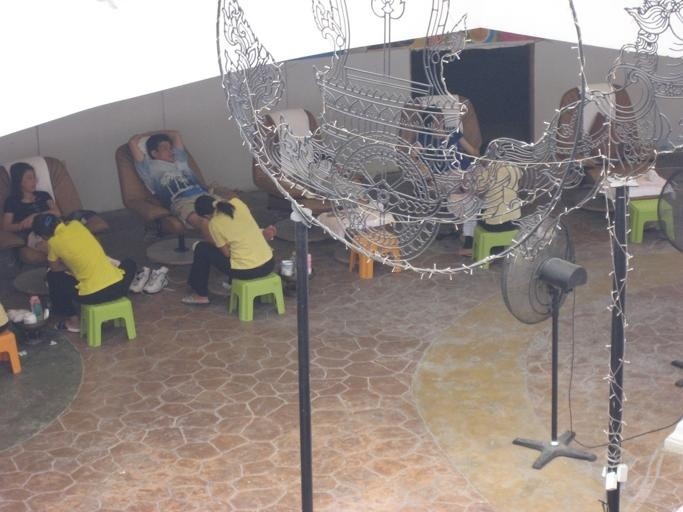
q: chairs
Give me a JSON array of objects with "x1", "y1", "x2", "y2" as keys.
[
  {"x1": 253, "y1": 108, "x2": 371, "y2": 244},
  {"x1": 115, "y1": 139, "x2": 239, "y2": 266},
  {"x1": 556, "y1": 83, "x2": 658, "y2": 213},
  {"x1": 395, "y1": 94, "x2": 498, "y2": 236},
  {"x1": 0, "y1": 157, "x2": 112, "y2": 294}
]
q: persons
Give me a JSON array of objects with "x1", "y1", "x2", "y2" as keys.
[
  {"x1": 1, "y1": 162, "x2": 64, "y2": 254},
  {"x1": 128, "y1": 130, "x2": 277, "y2": 241},
  {"x1": 181, "y1": 195, "x2": 275, "y2": 304},
  {"x1": 411, "y1": 104, "x2": 524, "y2": 257},
  {"x1": 0, "y1": 301, "x2": 27, "y2": 357},
  {"x1": 31, "y1": 213, "x2": 138, "y2": 332}
]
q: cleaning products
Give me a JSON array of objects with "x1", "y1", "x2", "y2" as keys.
[{"x1": 29, "y1": 294, "x2": 42, "y2": 320}]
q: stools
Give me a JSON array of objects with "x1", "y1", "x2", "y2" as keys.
[
  {"x1": 228, "y1": 270, "x2": 287, "y2": 323},
  {"x1": 624, "y1": 195, "x2": 677, "y2": 242},
  {"x1": 470, "y1": 226, "x2": 527, "y2": 272},
  {"x1": 0, "y1": 331, "x2": 21, "y2": 376},
  {"x1": 345, "y1": 231, "x2": 404, "y2": 281},
  {"x1": 79, "y1": 294, "x2": 138, "y2": 349}
]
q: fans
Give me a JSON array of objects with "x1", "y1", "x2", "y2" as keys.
[{"x1": 497, "y1": 208, "x2": 600, "y2": 471}]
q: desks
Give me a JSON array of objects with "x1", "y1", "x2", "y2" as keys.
[{"x1": 279, "y1": 267, "x2": 314, "y2": 297}]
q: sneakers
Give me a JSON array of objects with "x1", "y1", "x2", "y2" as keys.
[{"x1": 128, "y1": 264, "x2": 169, "y2": 294}]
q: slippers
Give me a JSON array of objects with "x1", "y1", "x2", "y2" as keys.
[
  {"x1": 181, "y1": 294, "x2": 212, "y2": 304},
  {"x1": 55, "y1": 319, "x2": 82, "y2": 334}
]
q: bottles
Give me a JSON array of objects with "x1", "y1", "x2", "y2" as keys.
[
  {"x1": 22, "y1": 296, "x2": 49, "y2": 324},
  {"x1": 280, "y1": 250, "x2": 311, "y2": 277}
]
